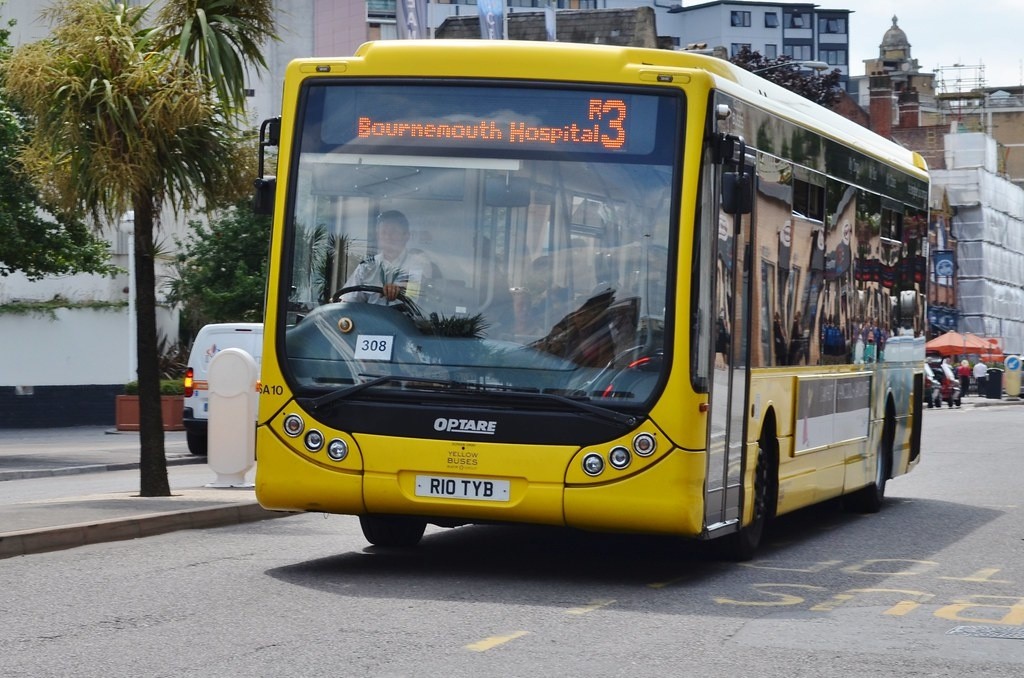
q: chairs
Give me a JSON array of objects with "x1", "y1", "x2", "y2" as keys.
[{"x1": 409, "y1": 250, "x2": 668, "y2": 352}]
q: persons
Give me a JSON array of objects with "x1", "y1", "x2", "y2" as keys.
[
  {"x1": 334, "y1": 209, "x2": 452, "y2": 321},
  {"x1": 713, "y1": 301, "x2": 922, "y2": 384},
  {"x1": 923, "y1": 353, "x2": 989, "y2": 411}
]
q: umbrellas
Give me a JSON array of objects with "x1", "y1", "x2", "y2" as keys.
[{"x1": 921, "y1": 327, "x2": 1002, "y2": 357}]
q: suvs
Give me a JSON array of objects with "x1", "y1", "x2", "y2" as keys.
[{"x1": 183, "y1": 322, "x2": 265, "y2": 459}]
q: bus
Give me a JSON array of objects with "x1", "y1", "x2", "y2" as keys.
[{"x1": 252, "y1": 39, "x2": 933, "y2": 552}]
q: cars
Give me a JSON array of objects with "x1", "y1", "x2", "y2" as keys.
[
  {"x1": 922, "y1": 363, "x2": 942, "y2": 408},
  {"x1": 928, "y1": 363, "x2": 962, "y2": 407}
]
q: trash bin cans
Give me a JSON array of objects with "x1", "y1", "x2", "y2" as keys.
[{"x1": 986, "y1": 367, "x2": 1005, "y2": 399}]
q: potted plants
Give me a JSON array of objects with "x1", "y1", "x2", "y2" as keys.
[{"x1": 115, "y1": 380, "x2": 185, "y2": 431}]
